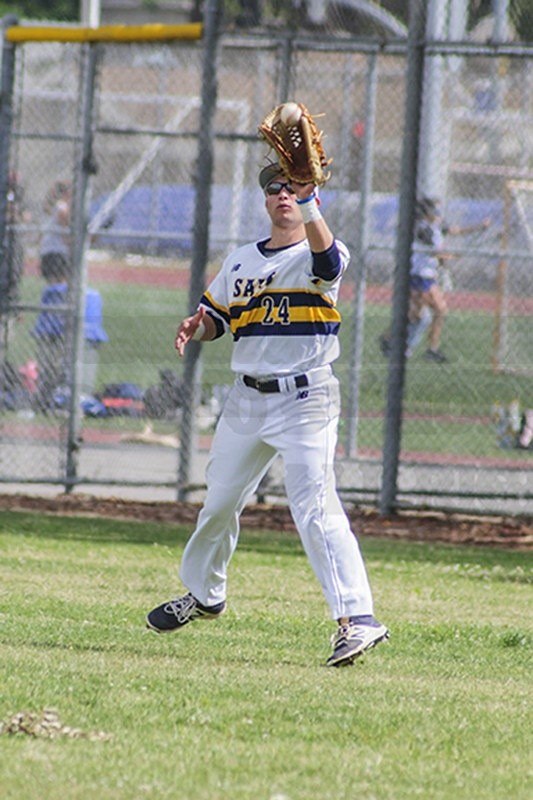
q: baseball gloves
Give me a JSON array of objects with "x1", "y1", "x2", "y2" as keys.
[{"x1": 259, "y1": 102, "x2": 325, "y2": 184}]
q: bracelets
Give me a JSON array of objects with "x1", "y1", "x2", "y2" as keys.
[{"x1": 192, "y1": 320, "x2": 205, "y2": 342}]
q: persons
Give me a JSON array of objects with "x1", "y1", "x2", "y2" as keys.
[
  {"x1": 30, "y1": 181, "x2": 107, "y2": 353},
  {"x1": 377, "y1": 196, "x2": 491, "y2": 366},
  {"x1": 143, "y1": 133, "x2": 390, "y2": 669}
]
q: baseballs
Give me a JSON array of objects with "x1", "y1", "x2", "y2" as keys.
[{"x1": 279, "y1": 103, "x2": 302, "y2": 124}]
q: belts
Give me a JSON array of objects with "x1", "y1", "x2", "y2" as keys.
[{"x1": 243, "y1": 372, "x2": 308, "y2": 393}]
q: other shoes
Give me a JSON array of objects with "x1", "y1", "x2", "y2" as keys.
[
  {"x1": 379, "y1": 331, "x2": 394, "y2": 357},
  {"x1": 430, "y1": 345, "x2": 449, "y2": 362}
]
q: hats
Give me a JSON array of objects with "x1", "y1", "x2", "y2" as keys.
[{"x1": 259, "y1": 160, "x2": 284, "y2": 189}]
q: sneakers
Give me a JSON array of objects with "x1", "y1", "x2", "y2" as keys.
[
  {"x1": 326, "y1": 614, "x2": 391, "y2": 665},
  {"x1": 146, "y1": 593, "x2": 228, "y2": 633}
]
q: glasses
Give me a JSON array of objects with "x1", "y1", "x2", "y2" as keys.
[{"x1": 265, "y1": 181, "x2": 295, "y2": 195}]
